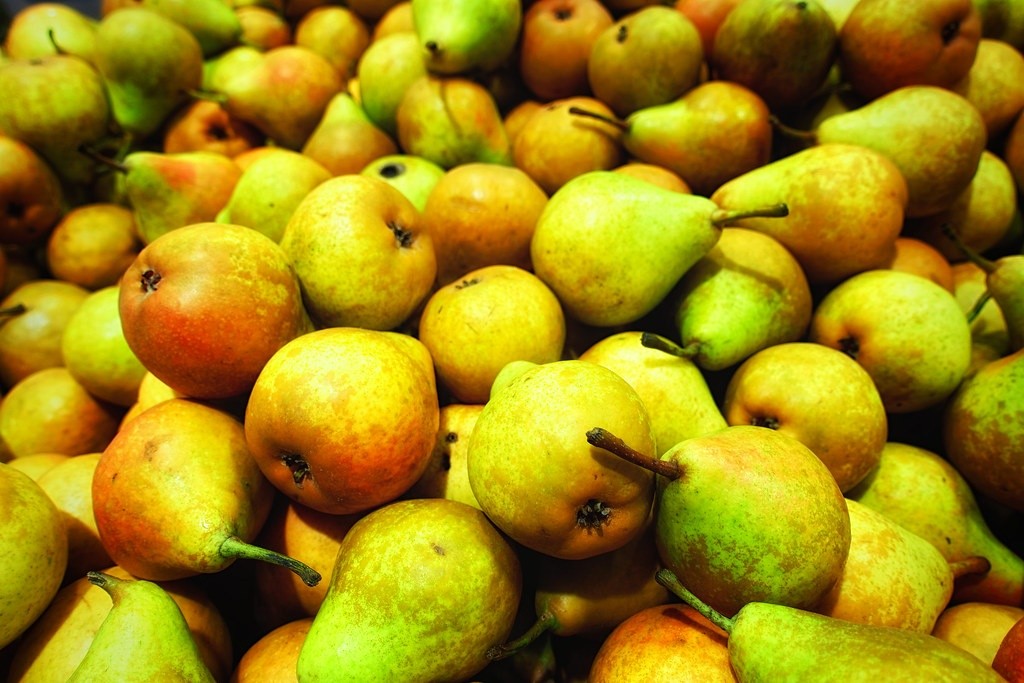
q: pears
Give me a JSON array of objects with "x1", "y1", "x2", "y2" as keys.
[{"x1": 0, "y1": 0, "x2": 1024, "y2": 683}]
